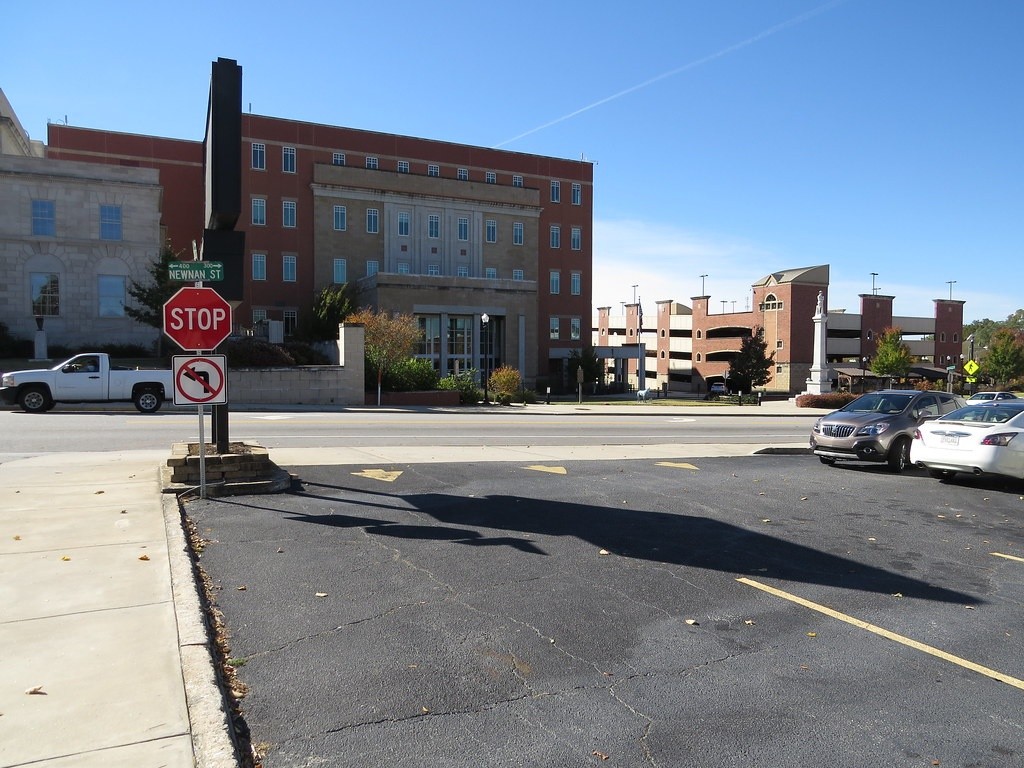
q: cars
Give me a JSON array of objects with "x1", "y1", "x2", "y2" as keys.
[
  {"x1": 965, "y1": 390, "x2": 1018, "y2": 406},
  {"x1": 809, "y1": 388, "x2": 969, "y2": 473},
  {"x1": 908, "y1": 397, "x2": 1024, "y2": 480}
]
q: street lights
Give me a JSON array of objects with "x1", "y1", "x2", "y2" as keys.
[{"x1": 482, "y1": 312, "x2": 490, "y2": 404}]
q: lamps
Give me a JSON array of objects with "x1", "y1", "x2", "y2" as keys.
[{"x1": 546, "y1": 385, "x2": 551, "y2": 394}]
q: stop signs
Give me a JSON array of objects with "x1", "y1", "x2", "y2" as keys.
[{"x1": 162, "y1": 287, "x2": 233, "y2": 351}]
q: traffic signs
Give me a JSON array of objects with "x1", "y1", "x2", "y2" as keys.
[{"x1": 168, "y1": 260, "x2": 225, "y2": 283}]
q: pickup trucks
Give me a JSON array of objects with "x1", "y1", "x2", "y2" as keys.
[{"x1": 0, "y1": 353, "x2": 174, "y2": 413}]
